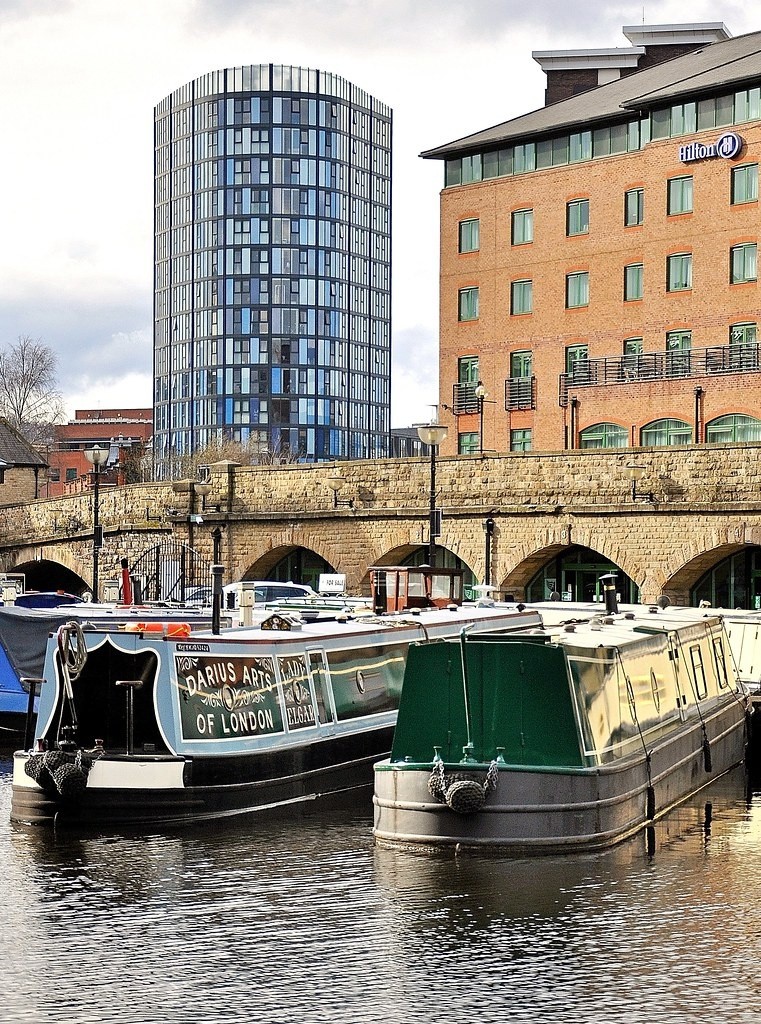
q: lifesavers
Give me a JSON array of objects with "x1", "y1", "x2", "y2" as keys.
[{"x1": 125, "y1": 623, "x2": 193, "y2": 639}]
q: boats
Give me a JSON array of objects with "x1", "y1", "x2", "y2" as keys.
[{"x1": 0, "y1": 574, "x2": 760, "y2": 857}]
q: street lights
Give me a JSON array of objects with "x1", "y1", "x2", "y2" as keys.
[
  {"x1": 418, "y1": 425, "x2": 449, "y2": 564},
  {"x1": 83, "y1": 442, "x2": 110, "y2": 608},
  {"x1": 475, "y1": 384, "x2": 489, "y2": 453}
]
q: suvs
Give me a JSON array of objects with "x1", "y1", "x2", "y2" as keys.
[
  {"x1": 183, "y1": 579, "x2": 317, "y2": 608},
  {"x1": 14, "y1": 591, "x2": 87, "y2": 609}
]
